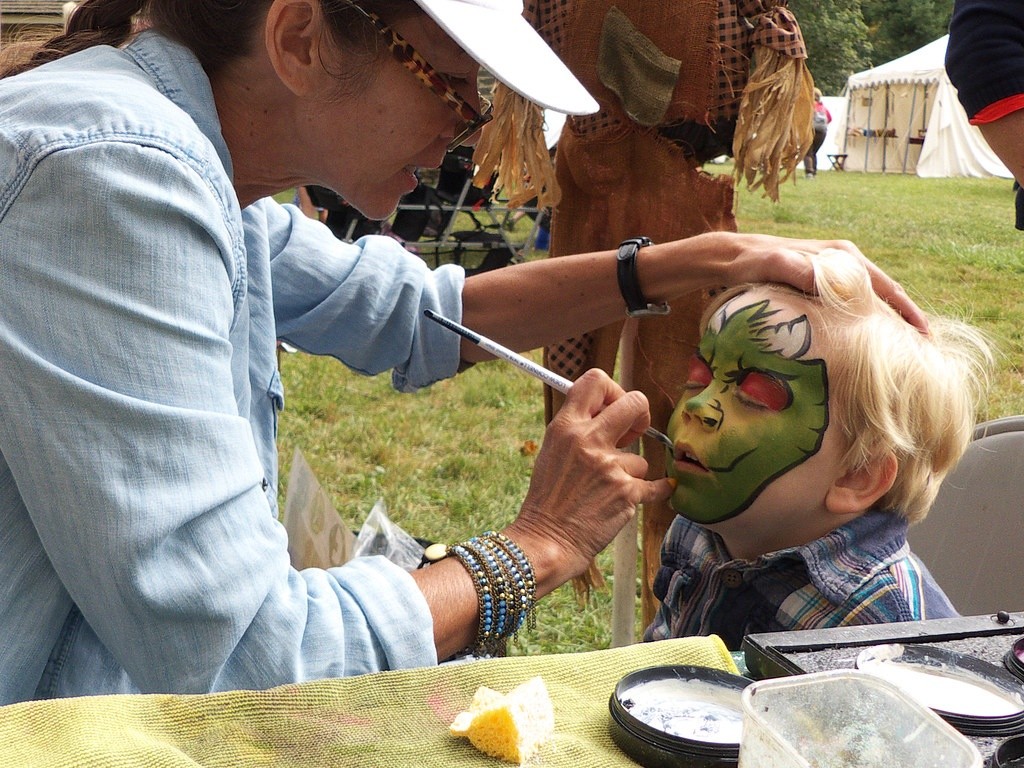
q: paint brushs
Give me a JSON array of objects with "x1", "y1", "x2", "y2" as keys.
[{"x1": 423, "y1": 308, "x2": 674, "y2": 450}]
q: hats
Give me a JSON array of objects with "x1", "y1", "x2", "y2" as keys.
[{"x1": 415, "y1": 0, "x2": 600, "y2": 117}]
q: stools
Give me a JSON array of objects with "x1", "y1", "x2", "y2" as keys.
[{"x1": 827, "y1": 154, "x2": 848, "y2": 171}]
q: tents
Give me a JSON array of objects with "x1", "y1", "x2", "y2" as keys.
[{"x1": 839, "y1": 33, "x2": 1014, "y2": 178}]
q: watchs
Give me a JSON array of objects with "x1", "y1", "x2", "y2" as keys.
[{"x1": 617, "y1": 237, "x2": 673, "y2": 319}]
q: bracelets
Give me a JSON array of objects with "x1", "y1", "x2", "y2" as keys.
[{"x1": 444, "y1": 530, "x2": 536, "y2": 660}]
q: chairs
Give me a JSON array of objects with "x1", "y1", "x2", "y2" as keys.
[{"x1": 905, "y1": 415, "x2": 1024, "y2": 617}]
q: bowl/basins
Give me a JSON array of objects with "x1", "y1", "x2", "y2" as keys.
[{"x1": 737, "y1": 669, "x2": 984, "y2": 768}]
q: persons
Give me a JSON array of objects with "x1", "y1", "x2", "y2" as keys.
[
  {"x1": 0, "y1": 0, "x2": 929, "y2": 708},
  {"x1": 804, "y1": 86, "x2": 832, "y2": 180},
  {"x1": 642, "y1": 249, "x2": 996, "y2": 652},
  {"x1": 944, "y1": 0, "x2": 1023, "y2": 234},
  {"x1": 294, "y1": 185, "x2": 382, "y2": 246}
]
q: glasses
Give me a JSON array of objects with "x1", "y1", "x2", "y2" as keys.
[{"x1": 349, "y1": 0, "x2": 495, "y2": 152}]
